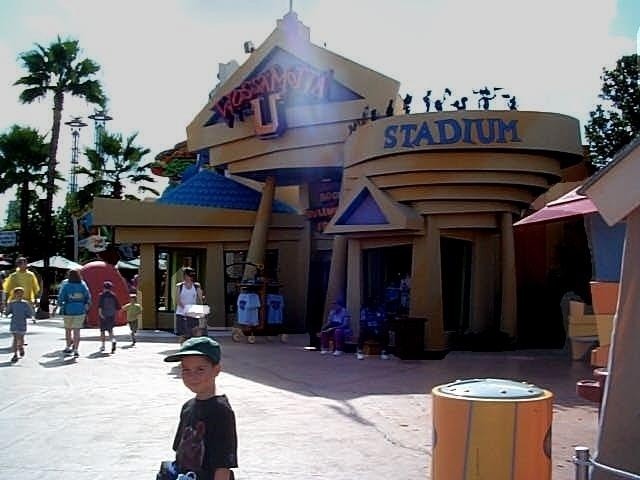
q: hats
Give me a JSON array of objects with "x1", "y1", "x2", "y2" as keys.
[
  {"x1": 165, "y1": 337, "x2": 221, "y2": 363},
  {"x1": 183, "y1": 268, "x2": 197, "y2": 277}
]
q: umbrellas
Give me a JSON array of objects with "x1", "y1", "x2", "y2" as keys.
[{"x1": 25, "y1": 252, "x2": 84, "y2": 305}]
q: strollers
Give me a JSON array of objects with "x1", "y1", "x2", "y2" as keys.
[{"x1": 181, "y1": 305, "x2": 212, "y2": 341}]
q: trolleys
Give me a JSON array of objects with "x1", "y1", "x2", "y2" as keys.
[{"x1": 220, "y1": 260, "x2": 291, "y2": 344}]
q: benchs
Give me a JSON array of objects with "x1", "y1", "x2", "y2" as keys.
[{"x1": 315, "y1": 332, "x2": 359, "y2": 353}]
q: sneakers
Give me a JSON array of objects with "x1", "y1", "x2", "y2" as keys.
[
  {"x1": 319, "y1": 349, "x2": 389, "y2": 360},
  {"x1": 11, "y1": 336, "x2": 138, "y2": 362}
]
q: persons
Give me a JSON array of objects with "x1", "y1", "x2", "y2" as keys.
[
  {"x1": 174, "y1": 264, "x2": 205, "y2": 347},
  {"x1": 154, "y1": 336, "x2": 240, "y2": 480},
  {"x1": 395, "y1": 270, "x2": 412, "y2": 315},
  {"x1": 1, "y1": 256, "x2": 42, "y2": 353},
  {"x1": 1, "y1": 286, "x2": 35, "y2": 362},
  {"x1": 119, "y1": 293, "x2": 141, "y2": 346},
  {"x1": 354, "y1": 294, "x2": 391, "y2": 362},
  {"x1": 95, "y1": 280, "x2": 122, "y2": 354},
  {"x1": 57, "y1": 268, "x2": 92, "y2": 357},
  {"x1": 318, "y1": 296, "x2": 353, "y2": 357}
]
